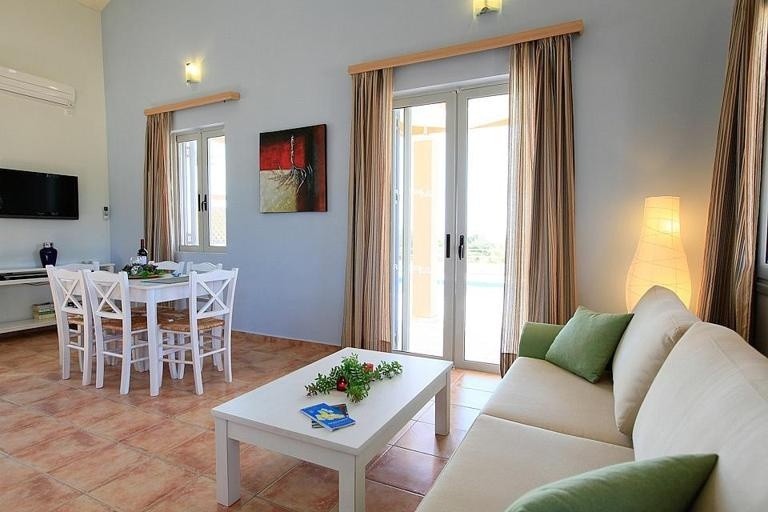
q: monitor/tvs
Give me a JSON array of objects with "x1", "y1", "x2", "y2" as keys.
[{"x1": 0, "y1": 168, "x2": 78, "y2": 220}]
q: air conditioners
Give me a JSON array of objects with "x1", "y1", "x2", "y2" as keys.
[{"x1": 0, "y1": 65, "x2": 75, "y2": 110}]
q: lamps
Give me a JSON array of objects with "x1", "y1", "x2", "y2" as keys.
[
  {"x1": 185, "y1": 61, "x2": 202, "y2": 84},
  {"x1": 625, "y1": 196, "x2": 692, "y2": 314},
  {"x1": 473, "y1": 0, "x2": 502, "y2": 17}
]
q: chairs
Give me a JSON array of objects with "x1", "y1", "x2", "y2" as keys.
[{"x1": 45, "y1": 261, "x2": 237, "y2": 397}]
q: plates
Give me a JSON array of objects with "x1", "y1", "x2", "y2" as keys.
[{"x1": 157, "y1": 269, "x2": 176, "y2": 273}]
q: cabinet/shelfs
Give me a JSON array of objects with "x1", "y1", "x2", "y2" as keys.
[{"x1": 0, "y1": 264, "x2": 115, "y2": 335}]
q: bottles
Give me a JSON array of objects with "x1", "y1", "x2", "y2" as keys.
[{"x1": 136, "y1": 238, "x2": 148, "y2": 264}]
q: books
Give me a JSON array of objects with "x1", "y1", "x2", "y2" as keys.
[
  {"x1": 310, "y1": 403, "x2": 350, "y2": 429},
  {"x1": 30, "y1": 301, "x2": 56, "y2": 323},
  {"x1": 299, "y1": 402, "x2": 355, "y2": 432}
]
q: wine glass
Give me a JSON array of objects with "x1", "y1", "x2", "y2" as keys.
[{"x1": 128, "y1": 256, "x2": 142, "y2": 277}]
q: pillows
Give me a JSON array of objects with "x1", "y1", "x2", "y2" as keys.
[
  {"x1": 631, "y1": 322, "x2": 767, "y2": 512},
  {"x1": 611, "y1": 286, "x2": 701, "y2": 438},
  {"x1": 503, "y1": 454, "x2": 717, "y2": 512},
  {"x1": 544, "y1": 305, "x2": 633, "y2": 384}
]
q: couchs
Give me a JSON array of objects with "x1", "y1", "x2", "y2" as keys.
[{"x1": 413, "y1": 322, "x2": 767, "y2": 512}]
